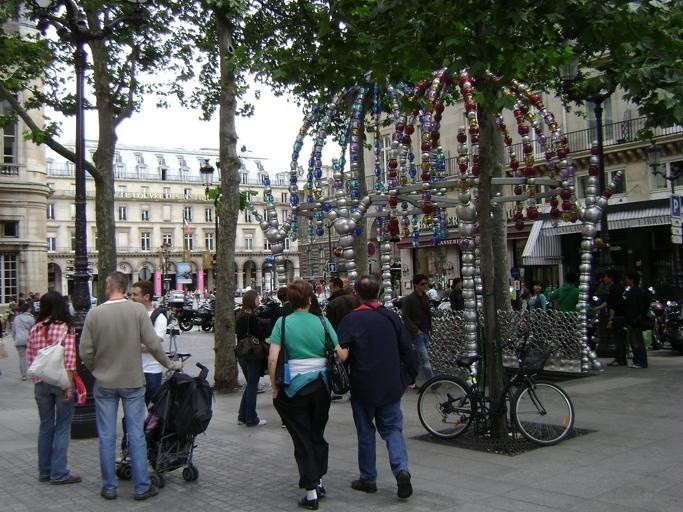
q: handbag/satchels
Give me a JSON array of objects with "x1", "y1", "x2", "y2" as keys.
[
  {"x1": 275, "y1": 316, "x2": 291, "y2": 387},
  {"x1": 27, "y1": 345, "x2": 72, "y2": 390},
  {"x1": 319, "y1": 315, "x2": 352, "y2": 396},
  {"x1": 234, "y1": 336, "x2": 263, "y2": 363}
]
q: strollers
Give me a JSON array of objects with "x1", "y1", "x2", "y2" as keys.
[{"x1": 115, "y1": 363, "x2": 211, "y2": 489}]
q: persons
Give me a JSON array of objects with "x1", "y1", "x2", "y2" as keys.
[
  {"x1": 119, "y1": 278, "x2": 168, "y2": 457},
  {"x1": 24, "y1": 291, "x2": 86, "y2": 487},
  {"x1": 78, "y1": 272, "x2": 184, "y2": 501},
  {"x1": 336, "y1": 269, "x2": 414, "y2": 501},
  {"x1": 2, "y1": 289, "x2": 84, "y2": 387},
  {"x1": 190, "y1": 288, "x2": 208, "y2": 303},
  {"x1": 266, "y1": 279, "x2": 347, "y2": 509},
  {"x1": 402, "y1": 271, "x2": 655, "y2": 390},
  {"x1": 234, "y1": 276, "x2": 362, "y2": 427}
]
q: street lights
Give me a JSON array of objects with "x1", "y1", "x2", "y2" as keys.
[
  {"x1": 559, "y1": 53, "x2": 619, "y2": 267},
  {"x1": 32, "y1": 0, "x2": 146, "y2": 331}
]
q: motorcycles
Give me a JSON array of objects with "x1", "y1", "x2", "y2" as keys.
[
  {"x1": 167, "y1": 289, "x2": 279, "y2": 332},
  {"x1": 651, "y1": 300, "x2": 683, "y2": 354}
]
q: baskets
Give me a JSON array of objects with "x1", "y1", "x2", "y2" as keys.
[{"x1": 520, "y1": 342, "x2": 553, "y2": 372}]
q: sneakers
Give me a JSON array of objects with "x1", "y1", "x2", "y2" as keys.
[
  {"x1": 258, "y1": 419, "x2": 268, "y2": 426},
  {"x1": 397, "y1": 471, "x2": 413, "y2": 499},
  {"x1": 607, "y1": 361, "x2": 626, "y2": 366},
  {"x1": 352, "y1": 479, "x2": 377, "y2": 493},
  {"x1": 239, "y1": 421, "x2": 245, "y2": 424},
  {"x1": 629, "y1": 365, "x2": 642, "y2": 368}
]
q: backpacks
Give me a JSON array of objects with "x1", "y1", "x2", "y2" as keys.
[{"x1": 175, "y1": 378, "x2": 217, "y2": 439}]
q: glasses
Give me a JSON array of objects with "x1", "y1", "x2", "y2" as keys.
[{"x1": 420, "y1": 282, "x2": 428, "y2": 286}]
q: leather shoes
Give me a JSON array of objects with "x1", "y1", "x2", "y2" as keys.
[
  {"x1": 317, "y1": 486, "x2": 326, "y2": 498},
  {"x1": 431, "y1": 384, "x2": 442, "y2": 390},
  {"x1": 299, "y1": 496, "x2": 319, "y2": 510},
  {"x1": 52, "y1": 475, "x2": 82, "y2": 485},
  {"x1": 102, "y1": 487, "x2": 118, "y2": 500},
  {"x1": 136, "y1": 485, "x2": 160, "y2": 501},
  {"x1": 40, "y1": 478, "x2": 51, "y2": 482}
]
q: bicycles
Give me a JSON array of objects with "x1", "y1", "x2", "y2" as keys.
[{"x1": 417, "y1": 331, "x2": 574, "y2": 446}]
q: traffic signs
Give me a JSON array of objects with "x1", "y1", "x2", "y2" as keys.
[{"x1": 328, "y1": 264, "x2": 335, "y2": 272}]
